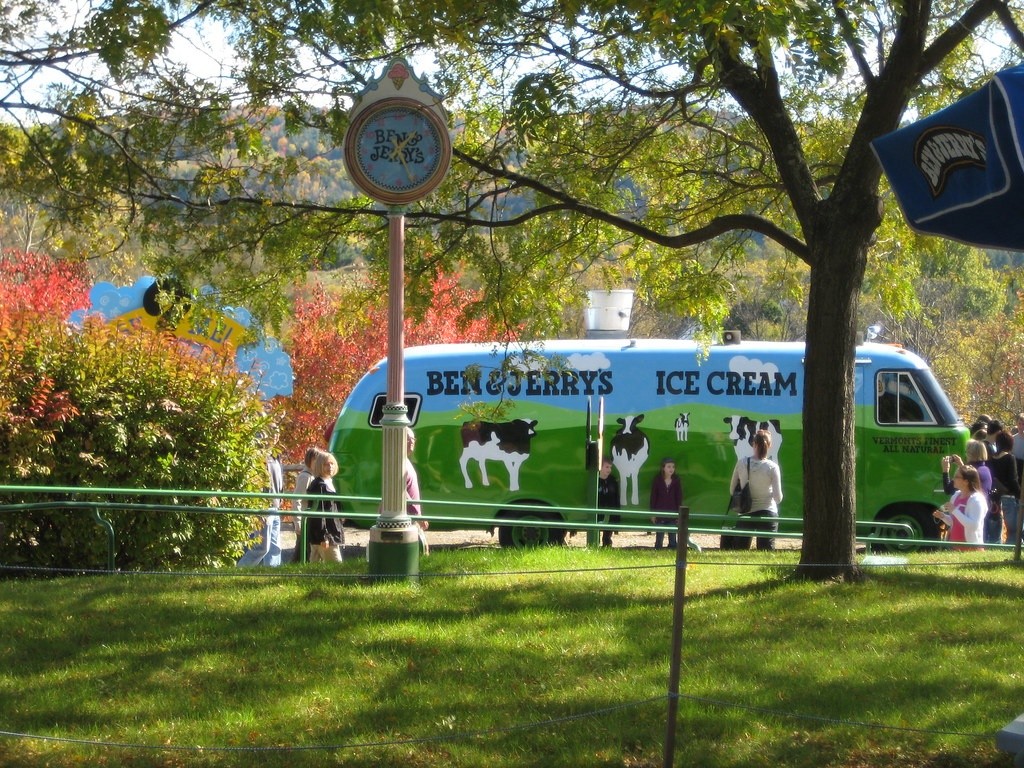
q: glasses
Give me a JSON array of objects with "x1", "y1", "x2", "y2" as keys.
[{"x1": 953, "y1": 475, "x2": 964, "y2": 480}]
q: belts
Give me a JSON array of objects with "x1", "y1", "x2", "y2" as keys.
[{"x1": 1001, "y1": 494, "x2": 1015, "y2": 498}]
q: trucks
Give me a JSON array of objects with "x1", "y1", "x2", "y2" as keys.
[{"x1": 324, "y1": 327, "x2": 972, "y2": 555}]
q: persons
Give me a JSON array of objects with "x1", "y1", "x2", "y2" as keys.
[
  {"x1": 650, "y1": 458, "x2": 684, "y2": 548},
  {"x1": 237, "y1": 421, "x2": 283, "y2": 568},
  {"x1": 598, "y1": 456, "x2": 622, "y2": 547},
  {"x1": 291, "y1": 449, "x2": 345, "y2": 562},
  {"x1": 932, "y1": 413, "x2": 1024, "y2": 551},
  {"x1": 730, "y1": 429, "x2": 783, "y2": 551},
  {"x1": 377, "y1": 426, "x2": 431, "y2": 556}
]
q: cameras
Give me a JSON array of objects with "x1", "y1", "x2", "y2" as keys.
[
  {"x1": 949, "y1": 456, "x2": 956, "y2": 463},
  {"x1": 941, "y1": 505, "x2": 948, "y2": 512}
]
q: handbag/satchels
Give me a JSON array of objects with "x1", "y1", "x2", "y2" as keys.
[
  {"x1": 720, "y1": 526, "x2": 736, "y2": 549},
  {"x1": 729, "y1": 457, "x2": 752, "y2": 514}
]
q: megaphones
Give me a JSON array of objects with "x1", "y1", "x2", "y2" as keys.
[{"x1": 719, "y1": 330, "x2": 741, "y2": 346}]
